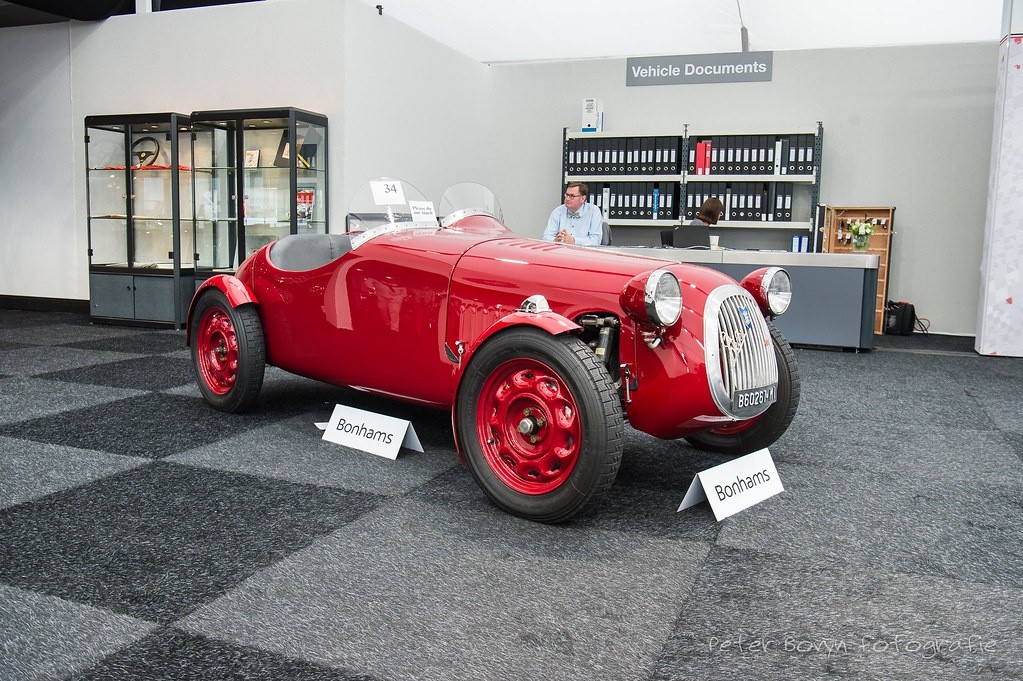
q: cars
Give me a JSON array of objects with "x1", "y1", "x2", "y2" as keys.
[{"x1": 186, "y1": 176, "x2": 802, "y2": 525}]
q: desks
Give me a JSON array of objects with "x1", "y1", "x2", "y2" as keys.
[{"x1": 602, "y1": 245, "x2": 882, "y2": 353}]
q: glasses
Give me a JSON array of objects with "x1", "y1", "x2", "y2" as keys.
[{"x1": 564, "y1": 194, "x2": 583, "y2": 199}]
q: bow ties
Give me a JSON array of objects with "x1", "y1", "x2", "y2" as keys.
[{"x1": 569, "y1": 212, "x2": 579, "y2": 218}]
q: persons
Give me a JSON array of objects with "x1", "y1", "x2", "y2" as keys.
[{"x1": 541, "y1": 181, "x2": 604, "y2": 248}]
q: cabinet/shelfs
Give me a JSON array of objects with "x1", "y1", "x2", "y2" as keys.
[
  {"x1": 812, "y1": 203, "x2": 896, "y2": 335},
  {"x1": 560, "y1": 119, "x2": 825, "y2": 249},
  {"x1": 83, "y1": 112, "x2": 216, "y2": 333},
  {"x1": 190, "y1": 106, "x2": 329, "y2": 291}
]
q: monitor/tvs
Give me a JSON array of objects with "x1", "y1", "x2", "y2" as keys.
[{"x1": 674, "y1": 225, "x2": 711, "y2": 250}]
